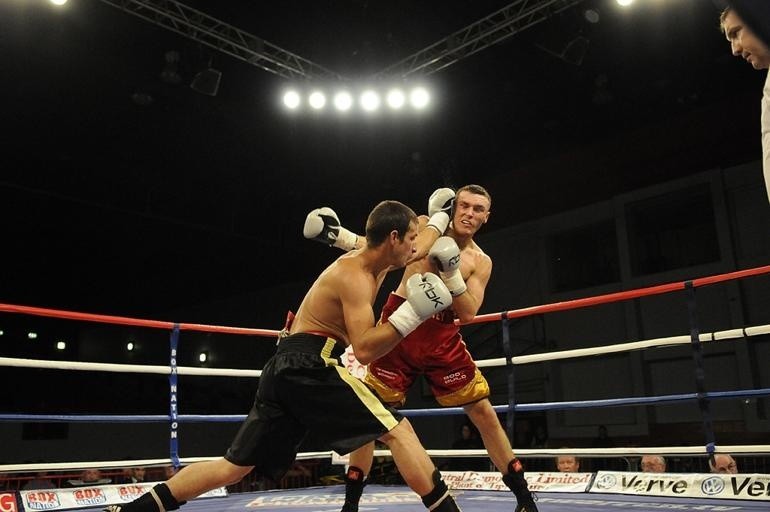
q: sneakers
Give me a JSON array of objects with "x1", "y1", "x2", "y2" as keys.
[{"x1": 515, "y1": 492, "x2": 538, "y2": 512}]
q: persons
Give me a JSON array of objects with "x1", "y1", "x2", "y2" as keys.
[
  {"x1": 22, "y1": 454, "x2": 407, "y2": 492},
  {"x1": 719, "y1": 0, "x2": 770, "y2": 205},
  {"x1": 708, "y1": 454, "x2": 738, "y2": 473},
  {"x1": 554, "y1": 446, "x2": 581, "y2": 472},
  {"x1": 301, "y1": 182, "x2": 541, "y2": 512},
  {"x1": 640, "y1": 455, "x2": 666, "y2": 472},
  {"x1": 513, "y1": 418, "x2": 556, "y2": 471},
  {"x1": 106, "y1": 199, "x2": 466, "y2": 512},
  {"x1": 451, "y1": 424, "x2": 482, "y2": 470}
]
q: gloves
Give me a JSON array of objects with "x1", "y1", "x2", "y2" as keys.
[
  {"x1": 303, "y1": 207, "x2": 356, "y2": 252},
  {"x1": 428, "y1": 236, "x2": 467, "y2": 297},
  {"x1": 425, "y1": 188, "x2": 456, "y2": 236},
  {"x1": 387, "y1": 272, "x2": 453, "y2": 338}
]
